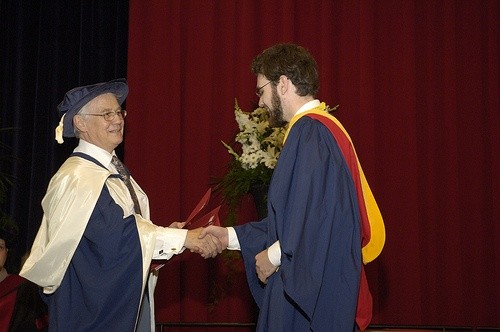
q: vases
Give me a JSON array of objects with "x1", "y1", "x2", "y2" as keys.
[{"x1": 245, "y1": 183, "x2": 272, "y2": 221}]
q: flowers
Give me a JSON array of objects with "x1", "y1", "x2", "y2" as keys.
[{"x1": 218, "y1": 96, "x2": 301, "y2": 202}]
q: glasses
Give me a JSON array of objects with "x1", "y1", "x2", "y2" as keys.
[
  {"x1": 75, "y1": 107, "x2": 127, "y2": 122},
  {"x1": 255, "y1": 79, "x2": 274, "y2": 98}
]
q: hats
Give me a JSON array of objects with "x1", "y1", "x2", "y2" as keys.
[{"x1": 52, "y1": 76, "x2": 131, "y2": 146}]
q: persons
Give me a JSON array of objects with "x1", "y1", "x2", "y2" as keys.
[
  {"x1": 190, "y1": 45, "x2": 386, "y2": 332},
  {"x1": 0, "y1": 212, "x2": 37, "y2": 332},
  {"x1": 21, "y1": 249, "x2": 49, "y2": 332},
  {"x1": 19, "y1": 81, "x2": 222, "y2": 332}
]
